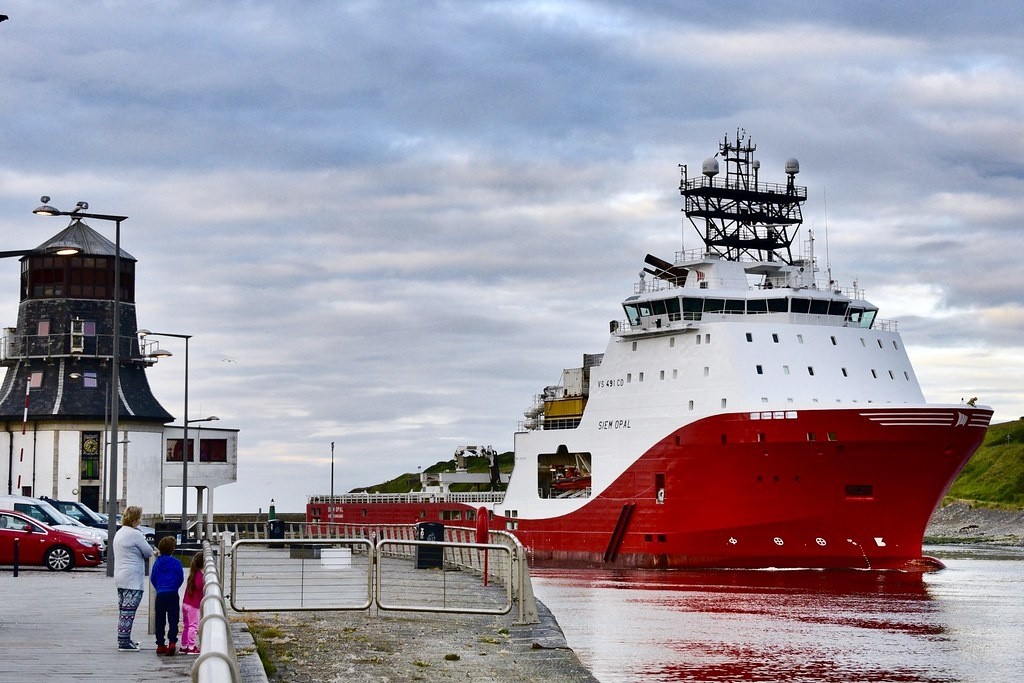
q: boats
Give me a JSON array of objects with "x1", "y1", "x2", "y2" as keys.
[
  {"x1": 552, "y1": 473, "x2": 591, "y2": 490},
  {"x1": 303, "y1": 126, "x2": 994, "y2": 571}
]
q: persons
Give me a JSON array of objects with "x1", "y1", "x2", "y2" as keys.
[
  {"x1": 179, "y1": 552, "x2": 205, "y2": 655},
  {"x1": 150, "y1": 536, "x2": 184, "y2": 657},
  {"x1": 112, "y1": 506, "x2": 153, "y2": 652},
  {"x1": 469, "y1": 484, "x2": 478, "y2": 492},
  {"x1": 786, "y1": 284, "x2": 791, "y2": 288},
  {"x1": 764, "y1": 281, "x2": 773, "y2": 289},
  {"x1": 960, "y1": 397, "x2": 977, "y2": 407}
]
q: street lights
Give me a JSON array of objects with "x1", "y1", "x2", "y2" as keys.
[
  {"x1": 136, "y1": 328, "x2": 220, "y2": 530},
  {"x1": 33, "y1": 206, "x2": 129, "y2": 577}
]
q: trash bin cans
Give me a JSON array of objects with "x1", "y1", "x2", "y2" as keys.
[
  {"x1": 414, "y1": 521, "x2": 446, "y2": 569},
  {"x1": 266, "y1": 519, "x2": 285, "y2": 548}
]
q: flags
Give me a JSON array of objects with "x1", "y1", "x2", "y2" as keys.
[{"x1": 695, "y1": 270, "x2": 705, "y2": 282}]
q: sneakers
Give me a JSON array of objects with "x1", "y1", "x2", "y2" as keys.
[
  {"x1": 131, "y1": 640, "x2": 141, "y2": 646},
  {"x1": 179, "y1": 647, "x2": 188, "y2": 654},
  {"x1": 118, "y1": 641, "x2": 140, "y2": 651},
  {"x1": 187, "y1": 646, "x2": 201, "y2": 654}
]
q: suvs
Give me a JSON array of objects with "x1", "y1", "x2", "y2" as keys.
[{"x1": 0, "y1": 495, "x2": 158, "y2": 571}]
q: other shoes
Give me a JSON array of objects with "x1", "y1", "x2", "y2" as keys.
[
  {"x1": 157, "y1": 645, "x2": 168, "y2": 653},
  {"x1": 166, "y1": 643, "x2": 176, "y2": 656}
]
